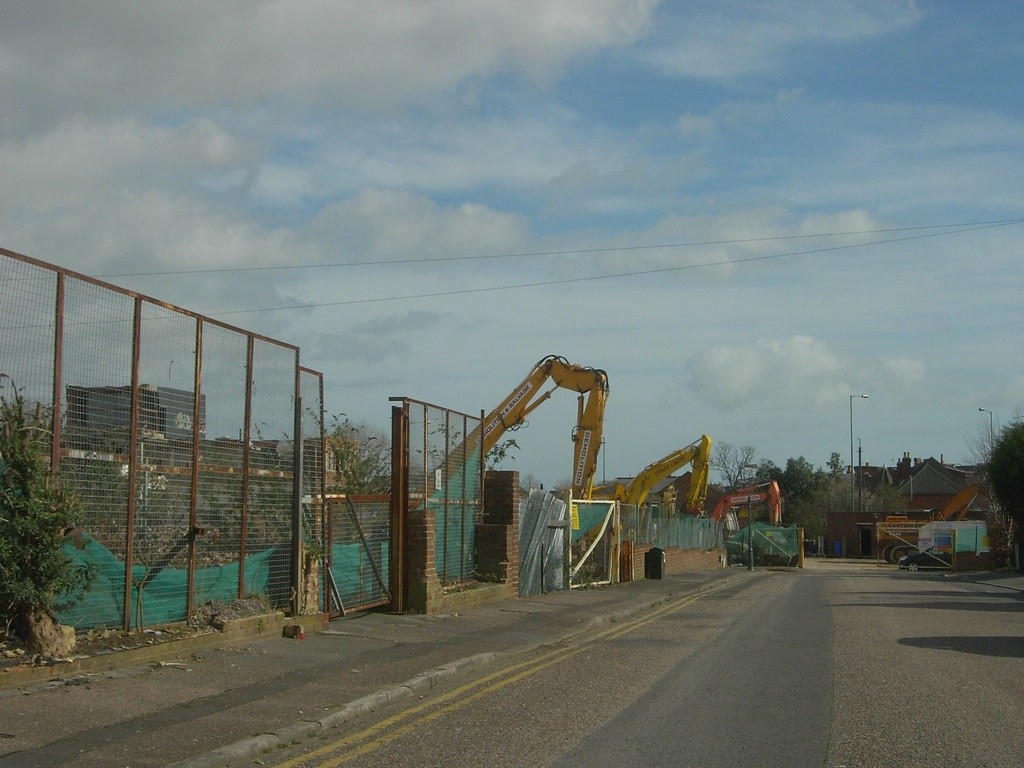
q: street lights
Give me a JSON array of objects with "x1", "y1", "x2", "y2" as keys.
[
  {"x1": 979, "y1": 407, "x2": 994, "y2": 455},
  {"x1": 849, "y1": 393, "x2": 869, "y2": 512}
]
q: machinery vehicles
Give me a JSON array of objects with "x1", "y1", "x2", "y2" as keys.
[
  {"x1": 589, "y1": 433, "x2": 712, "y2": 541},
  {"x1": 409, "y1": 354, "x2": 611, "y2": 511},
  {"x1": 711, "y1": 480, "x2": 783, "y2": 540},
  {"x1": 875, "y1": 483, "x2": 978, "y2": 564}
]
